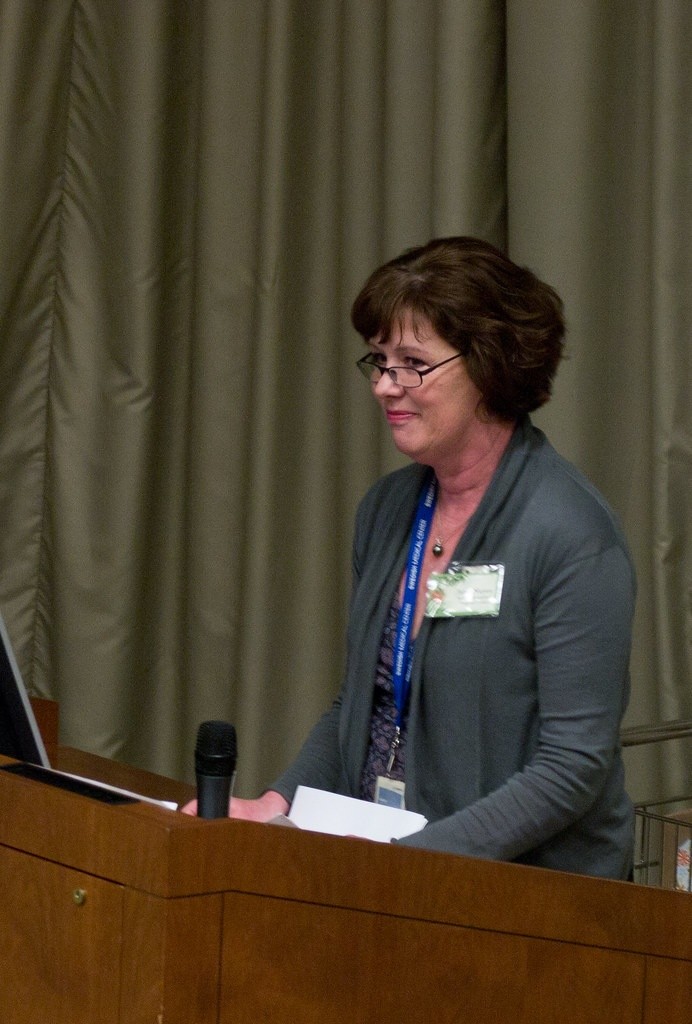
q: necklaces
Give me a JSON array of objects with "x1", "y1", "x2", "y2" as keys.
[{"x1": 432, "y1": 506, "x2": 466, "y2": 556}]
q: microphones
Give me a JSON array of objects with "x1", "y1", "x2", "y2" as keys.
[{"x1": 194, "y1": 720, "x2": 239, "y2": 820}]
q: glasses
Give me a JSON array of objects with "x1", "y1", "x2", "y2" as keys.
[{"x1": 354, "y1": 352, "x2": 460, "y2": 388}]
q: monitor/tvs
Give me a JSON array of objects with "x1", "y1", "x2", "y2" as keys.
[{"x1": 0, "y1": 620, "x2": 50, "y2": 770}]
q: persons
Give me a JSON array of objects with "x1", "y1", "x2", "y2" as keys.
[{"x1": 181, "y1": 237, "x2": 636, "y2": 882}]
